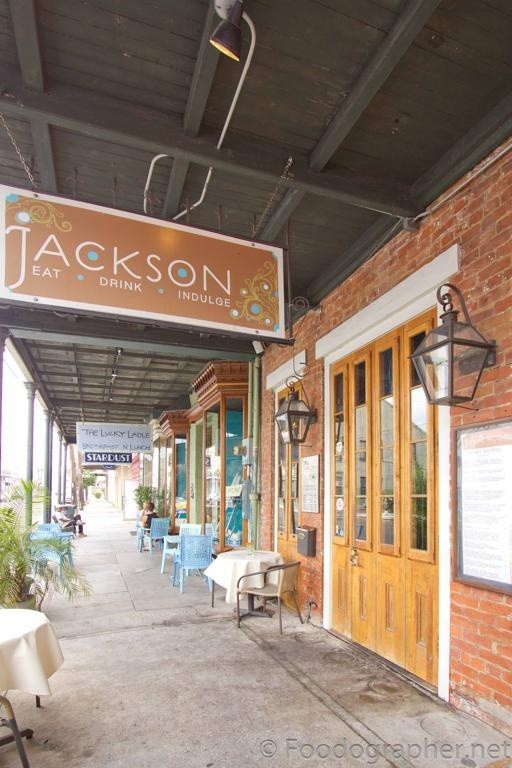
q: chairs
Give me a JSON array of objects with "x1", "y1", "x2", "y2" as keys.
[
  {"x1": 2, "y1": 503, "x2": 83, "y2": 768},
  {"x1": 129, "y1": 485, "x2": 305, "y2": 634}
]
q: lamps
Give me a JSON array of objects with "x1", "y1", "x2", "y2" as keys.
[
  {"x1": 409, "y1": 279, "x2": 497, "y2": 415},
  {"x1": 274, "y1": 373, "x2": 318, "y2": 448},
  {"x1": 207, "y1": 0, "x2": 247, "y2": 64}
]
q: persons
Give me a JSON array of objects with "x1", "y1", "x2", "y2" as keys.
[
  {"x1": 140, "y1": 501, "x2": 164, "y2": 544},
  {"x1": 55, "y1": 505, "x2": 87, "y2": 537}
]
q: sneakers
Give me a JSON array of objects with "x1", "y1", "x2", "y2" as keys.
[
  {"x1": 78, "y1": 533, "x2": 88, "y2": 538},
  {"x1": 76, "y1": 520, "x2": 86, "y2": 526}
]
